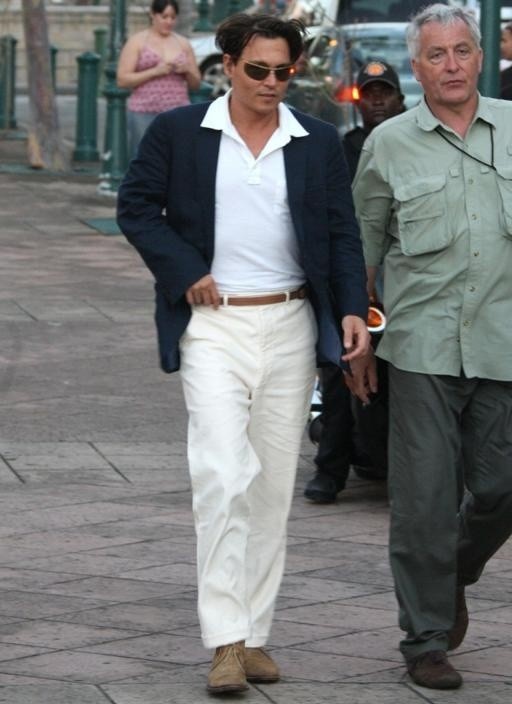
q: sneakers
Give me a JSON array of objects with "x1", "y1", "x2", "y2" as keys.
[
  {"x1": 444, "y1": 581, "x2": 469, "y2": 652},
  {"x1": 303, "y1": 461, "x2": 351, "y2": 504},
  {"x1": 405, "y1": 646, "x2": 463, "y2": 689},
  {"x1": 240, "y1": 643, "x2": 282, "y2": 684},
  {"x1": 207, "y1": 639, "x2": 249, "y2": 695}
]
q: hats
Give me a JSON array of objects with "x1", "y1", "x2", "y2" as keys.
[{"x1": 356, "y1": 61, "x2": 400, "y2": 91}]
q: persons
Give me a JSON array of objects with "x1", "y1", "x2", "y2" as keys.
[
  {"x1": 116, "y1": 12, "x2": 379, "y2": 699},
  {"x1": 338, "y1": 2, "x2": 512, "y2": 694},
  {"x1": 115, "y1": 2, "x2": 203, "y2": 161},
  {"x1": 496, "y1": 23, "x2": 512, "y2": 102},
  {"x1": 300, "y1": 59, "x2": 409, "y2": 504}
]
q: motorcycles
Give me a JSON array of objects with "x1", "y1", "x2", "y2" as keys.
[{"x1": 303, "y1": 259, "x2": 389, "y2": 481}]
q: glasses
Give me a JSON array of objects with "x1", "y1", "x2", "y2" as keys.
[{"x1": 229, "y1": 53, "x2": 292, "y2": 82}]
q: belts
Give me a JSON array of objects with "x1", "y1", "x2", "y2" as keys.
[{"x1": 215, "y1": 287, "x2": 307, "y2": 306}]
